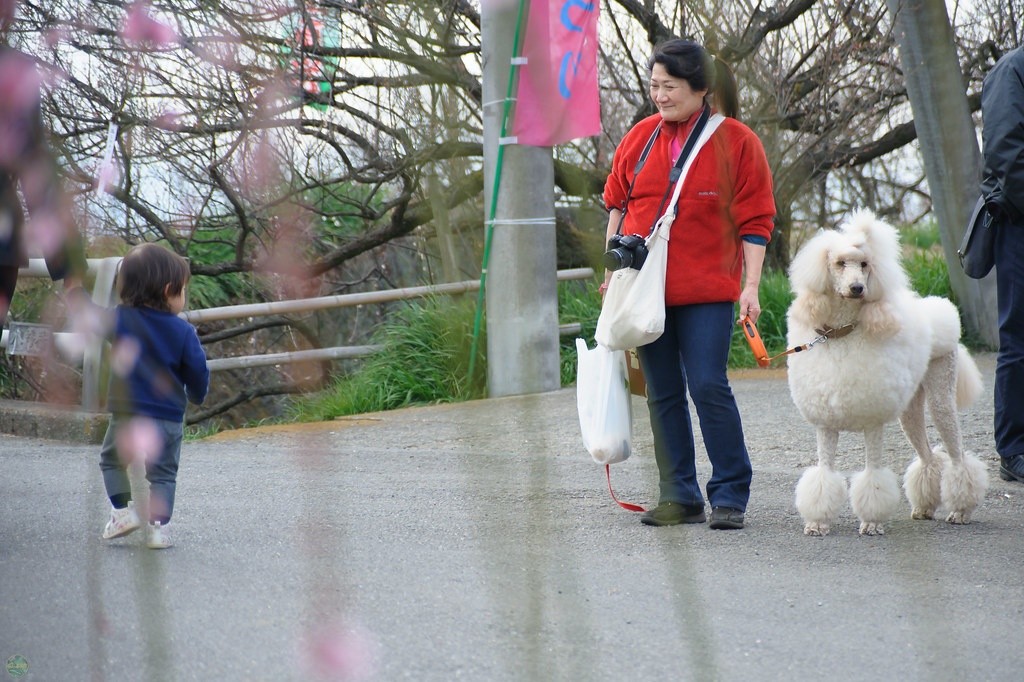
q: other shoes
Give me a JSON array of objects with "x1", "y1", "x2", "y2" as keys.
[{"x1": 1000, "y1": 454, "x2": 1024, "y2": 486}]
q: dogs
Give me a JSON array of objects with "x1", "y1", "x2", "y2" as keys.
[{"x1": 785, "y1": 204, "x2": 989, "y2": 536}]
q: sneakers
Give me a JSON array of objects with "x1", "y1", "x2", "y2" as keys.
[
  {"x1": 708, "y1": 503, "x2": 745, "y2": 530},
  {"x1": 145, "y1": 520, "x2": 173, "y2": 548},
  {"x1": 103, "y1": 501, "x2": 142, "y2": 539},
  {"x1": 641, "y1": 499, "x2": 707, "y2": 526}
]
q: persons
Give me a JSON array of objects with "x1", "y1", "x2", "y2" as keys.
[
  {"x1": 0, "y1": 33, "x2": 89, "y2": 338},
  {"x1": 603, "y1": 38, "x2": 777, "y2": 530},
  {"x1": 979, "y1": 42, "x2": 1024, "y2": 483},
  {"x1": 70, "y1": 242, "x2": 209, "y2": 549}
]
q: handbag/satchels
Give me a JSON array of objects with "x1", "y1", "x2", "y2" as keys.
[
  {"x1": 594, "y1": 214, "x2": 676, "y2": 351},
  {"x1": 575, "y1": 336, "x2": 632, "y2": 464},
  {"x1": 957, "y1": 195, "x2": 997, "y2": 279}
]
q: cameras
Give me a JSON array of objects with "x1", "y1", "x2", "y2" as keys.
[{"x1": 602, "y1": 233, "x2": 650, "y2": 272}]
